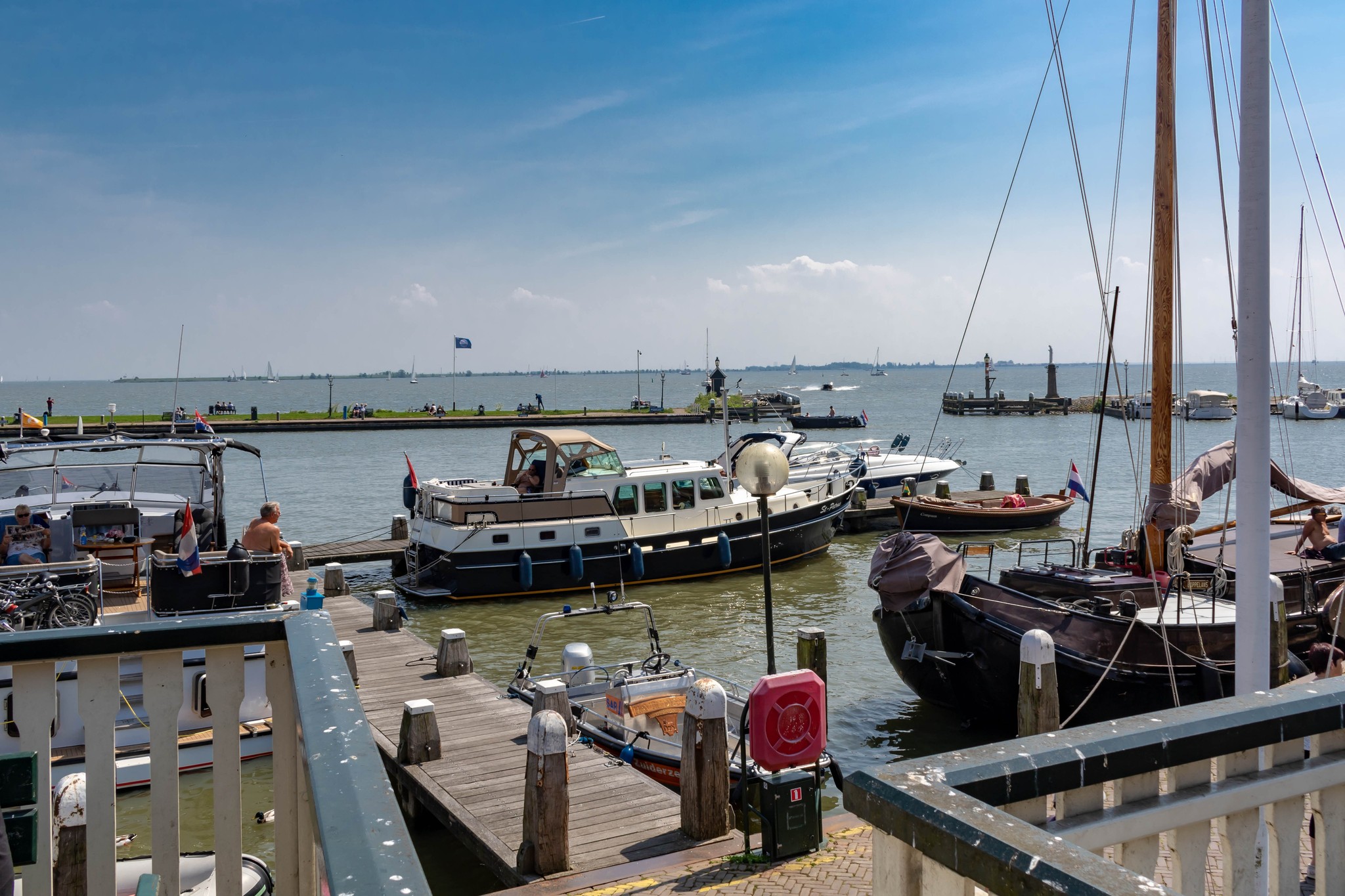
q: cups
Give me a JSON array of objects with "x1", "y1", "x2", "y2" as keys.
[
  {"x1": 107, "y1": 538, "x2": 114, "y2": 543},
  {"x1": 103, "y1": 538, "x2": 110, "y2": 543}
]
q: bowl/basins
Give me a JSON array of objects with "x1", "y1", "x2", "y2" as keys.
[{"x1": 123, "y1": 537, "x2": 135, "y2": 543}]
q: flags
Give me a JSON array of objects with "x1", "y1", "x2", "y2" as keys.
[
  {"x1": 455, "y1": 337, "x2": 472, "y2": 350},
  {"x1": 175, "y1": 500, "x2": 203, "y2": 579},
  {"x1": 22, "y1": 413, "x2": 43, "y2": 429},
  {"x1": 62, "y1": 475, "x2": 77, "y2": 489},
  {"x1": 1066, "y1": 461, "x2": 1091, "y2": 504}
]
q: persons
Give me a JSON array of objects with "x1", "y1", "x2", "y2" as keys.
[
  {"x1": 195, "y1": 409, "x2": 215, "y2": 434},
  {"x1": 0, "y1": 504, "x2": 59, "y2": 566},
  {"x1": 214, "y1": 401, "x2": 234, "y2": 415},
  {"x1": 513, "y1": 464, "x2": 540, "y2": 494},
  {"x1": 353, "y1": 403, "x2": 367, "y2": 418},
  {"x1": 632, "y1": 397, "x2": 653, "y2": 408},
  {"x1": 1284, "y1": 505, "x2": 1345, "y2": 560},
  {"x1": 46, "y1": 397, "x2": 55, "y2": 417},
  {"x1": 176, "y1": 407, "x2": 183, "y2": 420},
  {"x1": 241, "y1": 501, "x2": 293, "y2": 561},
  {"x1": 420, "y1": 403, "x2": 449, "y2": 416},
  {"x1": 517, "y1": 393, "x2": 544, "y2": 411}
]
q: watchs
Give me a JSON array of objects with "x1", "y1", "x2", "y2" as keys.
[{"x1": 528, "y1": 475, "x2": 532, "y2": 478}]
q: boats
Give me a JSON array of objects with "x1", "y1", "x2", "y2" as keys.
[
  {"x1": 890, "y1": 472, "x2": 1077, "y2": 531},
  {"x1": 1174, "y1": 390, "x2": 1233, "y2": 419},
  {"x1": 506, "y1": 590, "x2": 832, "y2": 817},
  {"x1": 820, "y1": 381, "x2": 834, "y2": 391},
  {"x1": 547, "y1": 373, "x2": 552, "y2": 376},
  {"x1": 543, "y1": 375, "x2": 548, "y2": 377},
  {"x1": 851, "y1": 432, "x2": 968, "y2": 498},
  {"x1": 789, "y1": 412, "x2": 862, "y2": 429},
  {"x1": 0, "y1": 407, "x2": 260, "y2": 551},
  {"x1": 1126, "y1": 389, "x2": 1151, "y2": 419},
  {"x1": 393, "y1": 428, "x2": 869, "y2": 600}
]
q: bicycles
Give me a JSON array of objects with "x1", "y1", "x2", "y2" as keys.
[{"x1": 0, "y1": 570, "x2": 98, "y2": 633}]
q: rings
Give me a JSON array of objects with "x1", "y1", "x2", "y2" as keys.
[{"x1": 7, "y1": 540, "x2": 8, "y2": 541}]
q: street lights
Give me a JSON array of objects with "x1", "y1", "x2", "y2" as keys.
[
  {"x1": 1123, "y1": 358, "x2": 1129, "y2": 399},
  {"x1": 735, "y1": 442, "x2": 790, "y2": 674},
  {"x1": 637, "y1": 349, "x2": 642, "y2": 413},
  {"x1": 660, "y1": 370, "x2": 666, "y2": 408},
  {"x1": 327, "y1": 374, "x2": 334, "y2": 418}
]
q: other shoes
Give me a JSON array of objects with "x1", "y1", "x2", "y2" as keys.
[{"x1": 357, "y1": 416, "x2": 359, "y2": 418}]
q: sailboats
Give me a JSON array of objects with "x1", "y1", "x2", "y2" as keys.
[
  {"x1": 870, "y1": 347, "x2": 888, "y2": 376},
  {"x1": 788, "y1": 354, "x2": 798, "y2": 374},
  {"x1": 866, "y1": 1, "x2": 1345, "y2": 725},
  {"x1": 387, "y1": 355, "x2": 446, "y2": 383},
  {"x1": 525, "y1": 364, "x2": 531, "y2": 377},
  {"x1": 1276, "y1": 203, "x2": 1340, "y2": 420},
  {"x1": 681, "y1": 360, "x2": 691, "y2": 375},
  {"x1": 227, "y1": 361, "x2": 280, "y2": 384},
  {"x1": 540, "y1": 370, "x2": 545, "y2": 378},
  {"x1": 840, "y1": 358, "x2": 849, "y2": 376},
  {"x1": 988, "y1": 357, "x2": 998, "y2": 372},
  {"x1": 700, "y1": 327, "x2": 712, "y2": 386}
]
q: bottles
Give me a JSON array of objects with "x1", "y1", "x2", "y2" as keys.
[
  {"x1": 81, "y1": 533, "x2": 86, "y2": 545},
  {"x1": 80, "y1": 525, "x2": 86, "y2": 543}
]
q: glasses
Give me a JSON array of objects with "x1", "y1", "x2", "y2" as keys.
[
  {"x1": 273, "y1": 512, "x2": 281, "y2": 515},
  {"x1": 16, "y1": 513, "x2": 29, "y2": 518},
  {"x1": 528, "y1": 468, "x2": 535, "y2": 471},
  {"x1": 1315, "y1": 513, "x2": 1327, "y2": 516}
]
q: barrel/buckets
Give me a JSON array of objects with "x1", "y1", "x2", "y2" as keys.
[{"x1": 1147, "y1": 571, "x2": 1170, "y2": 593}]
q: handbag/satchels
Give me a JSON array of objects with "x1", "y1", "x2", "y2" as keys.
[{"x1": 44, "y1": 412, "x2": 48, "y2": 416}]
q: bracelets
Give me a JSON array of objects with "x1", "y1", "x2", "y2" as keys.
[{"x1": 2, "y1": 542, "x2": 8, "y2": 546}]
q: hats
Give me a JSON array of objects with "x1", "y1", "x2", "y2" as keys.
[
  {"x1": 48, "y1": 397, "x2": 51, "y2": 399},
  {"x1": 556, "y1": 462, "x2": 559, "y2": 467},
  {"x1": 829, "y1": 406, "x2": 833, "y2": 407},
  {"x1": 356, "y1": 403, "x2": 358, "y2": 405}
]
